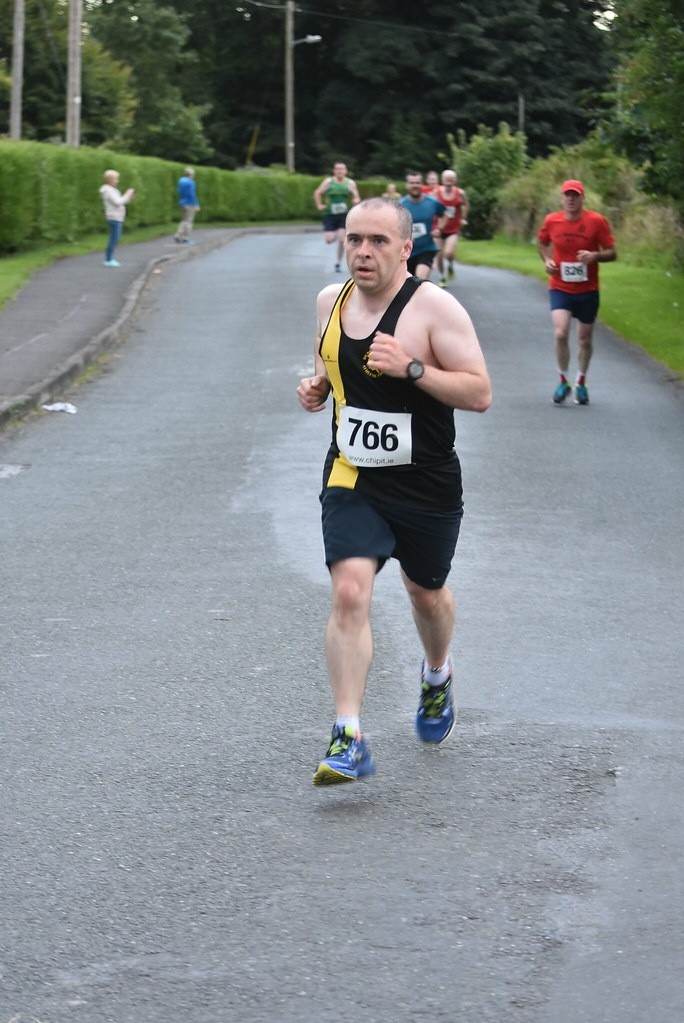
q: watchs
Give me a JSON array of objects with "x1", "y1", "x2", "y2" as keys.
[{"x1": 403, "y1": 359, "x2": 426, "y2": 383}]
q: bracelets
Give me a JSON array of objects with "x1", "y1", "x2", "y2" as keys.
[{"x1": 437, "y1": 227, "x2": 444, "y2": 233}]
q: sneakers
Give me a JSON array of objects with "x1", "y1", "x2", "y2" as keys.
[
  {"x1": 552, "y1": 383, "x2": 571, "y2": 404},
  {"x1": 313, "y1": 725, "x2": 376, "y2": 783},
  {"x1": 335, "y1": 265, "x2": 340, "y2": 272},
  {"x1": 575, "y1": 384, "x2": 589, "y2": 404},
  {"x1": 415, "y1": 653, "x2": 456, "y2": 745},
  {"x1": 438, "y1": 278, "x2": 447, "y2": 288},
  {"x1": 103, "y1": 259, "x2": 120, "y2": 268},
  {"x1": 448, "y1": 268, "x2": 455, "y2": 281}
]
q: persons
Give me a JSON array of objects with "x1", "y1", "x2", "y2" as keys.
[
  {"x1": 98, "y1": 168, "x2": 134, "y2": 267},
  {"x1": 380, "y1": 183, "x2": 402, "y2": 199},
  {"x1": 430, "y1": 169, "x2": 469, "y2": 288},
  {"x1": 173, "y1": 166, "x2": 202, "y2": 246},
  {"x1": 314, "y1": 162, "x2": 361, "y2": 273},
  {"x1": 398, "y1": 172, "x2": 448, "y2": 281},
  {"x1": 537, "y1": 179, "x2": 618, "y2": 404},
  {"x1": 422, "y1": 171, "x2": 440, "y2": 196},
  {"x1": 295, "y1": 196, "x2": 490, "y2": 785}
]
